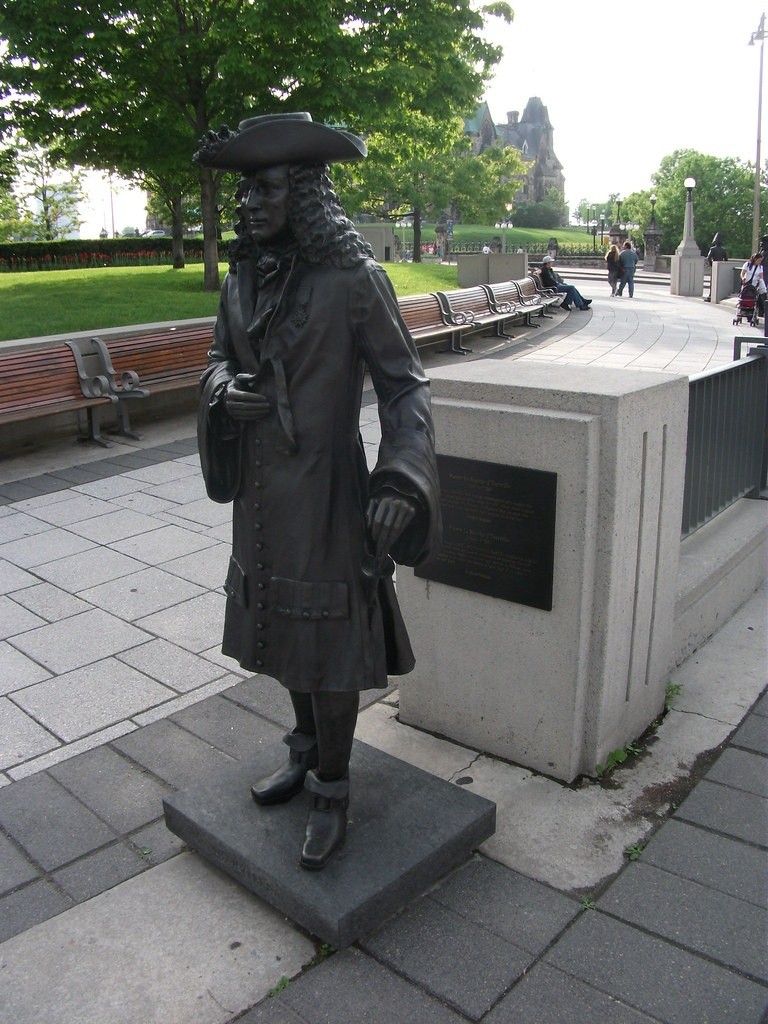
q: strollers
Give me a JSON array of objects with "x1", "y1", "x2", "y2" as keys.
[{"x1": 732, "y1": 280, "x2": 760, "y2": 327}]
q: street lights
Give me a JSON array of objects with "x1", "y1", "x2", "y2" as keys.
[
  {"x1": 494, "y1": 219, "x2": 515, "y2": 254},
  {"x1": 585, "y1": 204, "x2": 592, "y2": 234},
  {"x1": 395, "y1": 220, "x2": 413, "y2": 254},
  {"x1": 643, "y1": 193, "x2": 659, "y2": 231},
  {"x1": 749, "y1": 11, "x2": 768, "y2": 257},
  {"x1": 618, "y1": 220, "x2": 640, "y2": 243},
  {"x1": 675, "y1": 177, "x2": 702, "y2": 256},
  {"x1": 600, "y1": 213, "x2": 606, "y2": 246},
  {"x1": 590, "y1": 220, "x2": 598, "y2": 255},
  {"x1": 614, "y1": 196, "x2": 623, "y2": 226},
  {"x1": 592, "y1": 205, "x2": 598, "y2": 221}
]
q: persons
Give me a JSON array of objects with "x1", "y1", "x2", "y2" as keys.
[
  {"x1": 707, "y1": 232, "x2": 728, "y2": 296},
  {"x1": 539, "y1": 256, "x2": 592, "y2": 311},
  {"x1": 195, "y1": 111, "x2": 440, "y2": 867},
  {"x1": 605, "y1": 242, "x2": 639, "y2": 298},
  {"x1": 516, "y1": 247, "x2": 523, "y2": 253},
  {"x1": 483, "y1": 244, "x2": 494, "y2": 254},
  {"x1": 736, "y1": 254, "x2": 764, "y2": 317},
  {"x1": 756, "y1": 234, "x2": 768, "y2": 287},
  {"x1": 420, "y1": 243, "x2": 440, "y2": 257}
]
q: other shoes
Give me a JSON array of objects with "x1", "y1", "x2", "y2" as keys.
[
  {"x1": 580, "y1": 304, "x2": 590, "y2": 310},
  {"x1": 582, "y1": 299, "x2": 592, "y2": 305},
  {"x1": 560, "y1": 303, "x2": 571, "y2": 311},
  {"x1": 747, "y1": 319, "x2": 751, "y2": 322}
]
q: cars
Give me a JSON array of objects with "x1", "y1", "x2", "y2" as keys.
[{"x1": 142, "y1": 230, "x2": 165, "y2": 238}]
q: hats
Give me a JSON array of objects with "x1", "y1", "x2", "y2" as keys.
[{"x1": 543, "y1": 256, "x2": 555, "y2": 264}]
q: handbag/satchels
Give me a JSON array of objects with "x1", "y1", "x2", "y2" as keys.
[
  {"x1": 743, "y1": 277, "x2": 752, "y2": 287},
  {"x1": 618, "y1": 268, "x2": 624, "y2": 278}
]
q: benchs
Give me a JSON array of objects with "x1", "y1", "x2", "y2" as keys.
[{"x1": 1, "y1": 267, "x2": 570, "y2": 447}]
